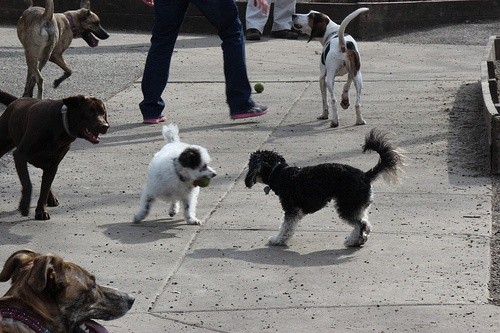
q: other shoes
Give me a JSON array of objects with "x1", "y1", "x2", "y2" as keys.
[
  {"x1": 246, "y1": 28, "x2": 261, "y2": 40},
  {"x1": 270, "y1": 30, "x2": 299, "y2": 39}
]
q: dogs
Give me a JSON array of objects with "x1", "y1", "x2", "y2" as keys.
[
  {"x1": 290, "y1": 7, "x2": 370, "y2": 128},
  {"x1": 10, "y1": 0, "x2": 111, "y2": 101},
  {"x1": 0, "y1": 89, "x2": 110, "y2": 221},
  {"x1": 244, "y1": 127, "x2": 414, "y2": 248},
  {"x1": 0, "y1": 249, "x2": 135, "y2": 333},
  {"x1": 133, "y1": 122, "x2": 218, "y2": 224}
]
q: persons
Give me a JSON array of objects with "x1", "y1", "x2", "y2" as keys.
[
  {"x1": 246, "y1": 0, "x2": 299, "y2": 40},
  {"x1": 139, "y1": 0, "x2": 268, "y2": 123}
]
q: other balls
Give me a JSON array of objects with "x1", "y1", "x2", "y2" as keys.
[{"x1": 254, "y1": 84, "x2": 264, "y2": 93}]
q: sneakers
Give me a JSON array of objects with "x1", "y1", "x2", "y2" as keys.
[
  {"x1": 232, "y1": 97, "x2": 267, "y2": 119},
  {"x1": 143, "y1": 113, "x2": 166, "y2": 123}
]
